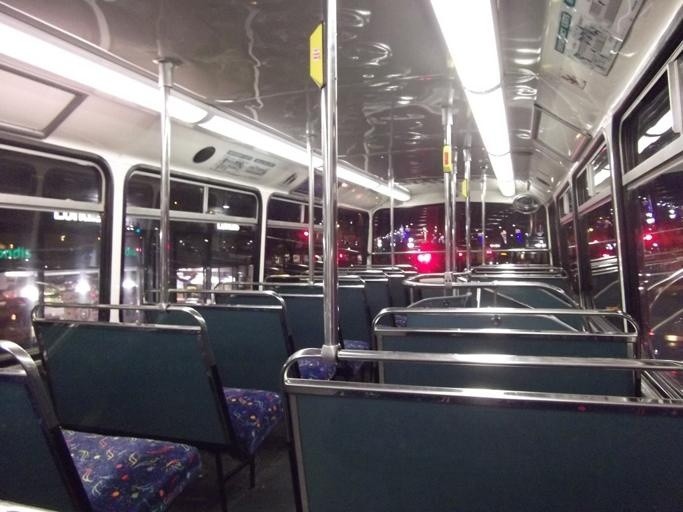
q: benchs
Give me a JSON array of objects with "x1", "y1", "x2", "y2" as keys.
[{"x1": 0, "y1": 266, "x2": 682, "y2": 512}]
